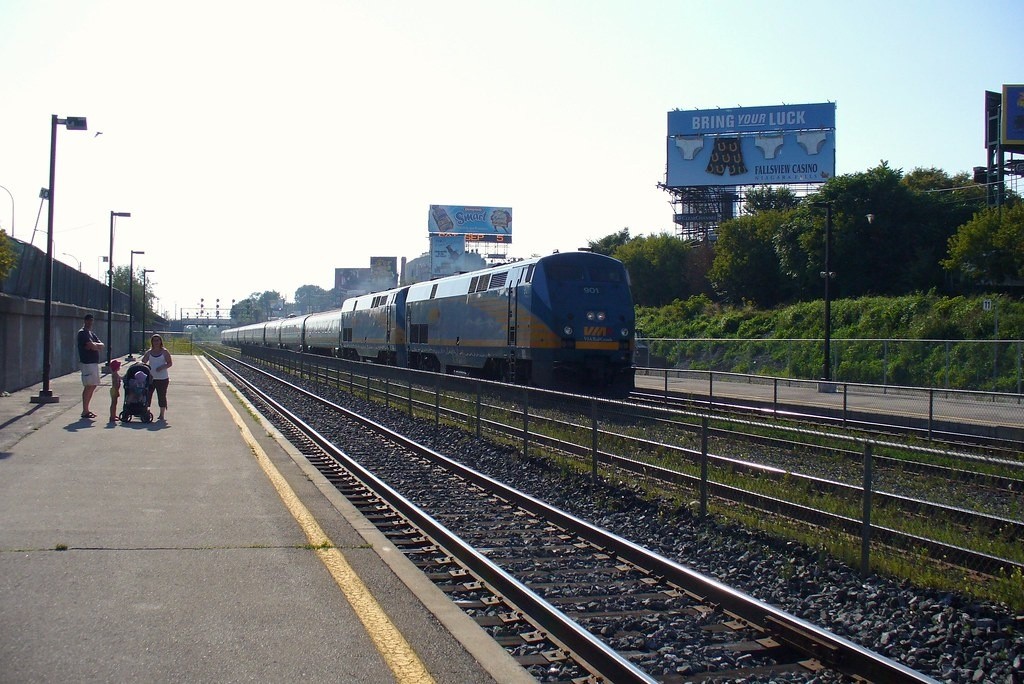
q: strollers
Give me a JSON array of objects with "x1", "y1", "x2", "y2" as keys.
[{"x1": 118, "y1": 362, "x2": 154, "y2": 424}]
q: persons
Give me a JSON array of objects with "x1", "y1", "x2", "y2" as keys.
[
  {"x1": 126, "y1": 371, "x2": 148, "y2": 407},
  {"x1": 109, "y1": 359, "x2": 124, "y2": 421},
  {"x1": 142, "y1": 334, "x2": 172, "y2": 420},
  {"x1": 77, "y1": 313, "x2": 105, "y2": 418}
]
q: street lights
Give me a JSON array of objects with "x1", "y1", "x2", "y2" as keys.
[
  {"x1": 62, "y1": 252, "x2": 82, "y2": 272},
  {"x1": 33, "y1": 228, "x2": 56, "y2": 259},
  {"x1": 811, "y1": 200, "x2": 840, "y2": 395},
  {"x1": 139, "y1": 268, "x2": 155, "y2": 355},
  {"x1": 124, "y1": 250, "x2": 145, "y2": 362},
  {"x1": 27, "y1": 115, "x2": 89, "y2": 404},
  {"x1": 102, "y1": 211, "x2": 131, "y2": 373}
]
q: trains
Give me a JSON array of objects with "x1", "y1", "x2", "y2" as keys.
[{"x1": 220, "y1": 250, "x2": 637, "y2": 405}]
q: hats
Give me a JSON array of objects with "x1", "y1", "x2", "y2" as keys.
[
  {"x1": 135, "y1": 371, "x2": 147, "y2": 383},
  {"x1": 84, "y1": 313, "x2": 93, "y2": 319},
  {"x1": 110, "y1": 360, "x2": 121, "y2": 369}
]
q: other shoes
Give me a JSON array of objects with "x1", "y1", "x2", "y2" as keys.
[
  {"x1": 157, "y1": 415, "x2": 164, "y2": 420},
  {"x1": 110, "y1": 416, "x2": 119, "y2": 421}
]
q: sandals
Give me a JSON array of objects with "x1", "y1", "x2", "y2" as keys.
[{"x1": 82, "y1": 411, "x2": 96, "y2": 417}]
